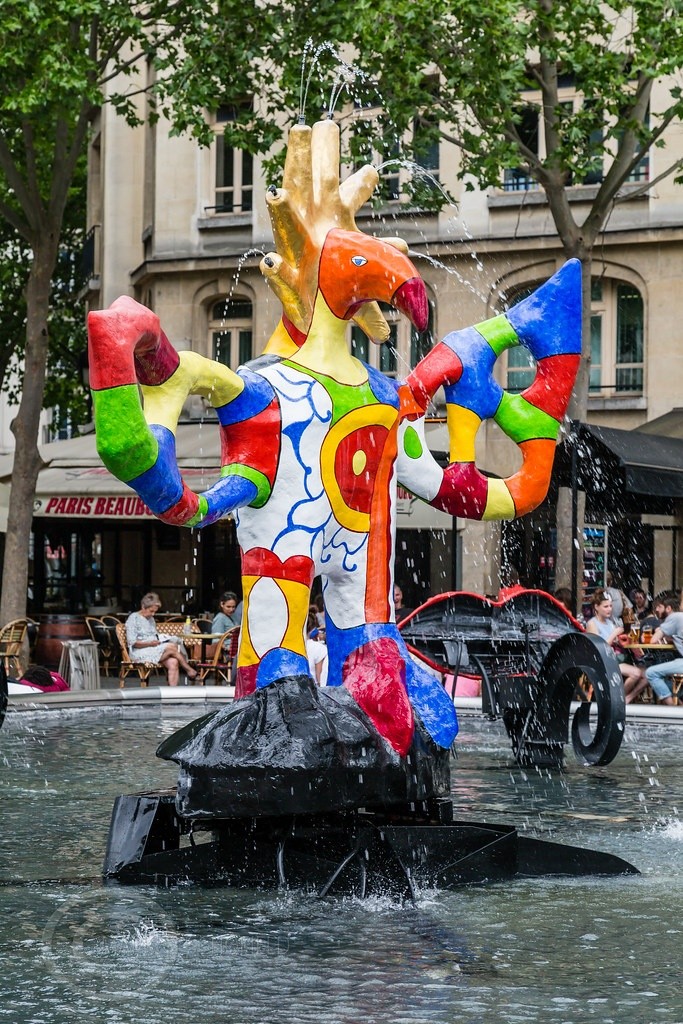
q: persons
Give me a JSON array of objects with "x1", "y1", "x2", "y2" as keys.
[
  {"x1": 125, "y1": 592, "x2": 201, "y2": 686},
  {"x1": 212, "y1": 584, "x2": 408, "y2": 687},
  {"x1": 555, "y1": 570, "x2": 683, "y2": 706},
  {"x1": 7, "y1": 666, "x2": 70, "y2": 693},
  {"x1": 498, "y1": 561, "x2": 527, "y2": 602}
]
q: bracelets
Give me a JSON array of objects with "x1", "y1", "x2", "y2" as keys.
[{"x1": 317, "y1": 682, "x2": 320, "y2": 686}]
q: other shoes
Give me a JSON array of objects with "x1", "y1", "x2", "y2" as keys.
[{"x1": 187, "y1": 670, "x2": 208, "y2": 681}]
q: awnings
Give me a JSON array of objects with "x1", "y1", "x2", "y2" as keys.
[
  {"x1": 33, "y1": 467, "x2": 465, "y2": 530},
  {"x1": 554, "y1": 420, "x2": 683, "y2": 495}
]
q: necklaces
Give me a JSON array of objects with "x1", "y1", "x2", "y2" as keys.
[{"x1": 596, "y1": 613, "x2": 606, "y2": 624}]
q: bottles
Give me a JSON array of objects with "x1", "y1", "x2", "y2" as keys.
[{"x1": 185, "y1": 615, "x2": 190, "y2": 635}]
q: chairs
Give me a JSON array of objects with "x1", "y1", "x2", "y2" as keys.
[
  {"x1": 0, "y1": 617, "x2": 29, "y2": 681},
  {"x1": 86, "y1": 612, "x2": 241, "y2": 688},
  {"x1": 577, "y1": 672, "x2": 683, "y2": 705}
]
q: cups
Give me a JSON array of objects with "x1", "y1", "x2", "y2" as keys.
[
  {"x1": 628, "y1": 624, "x2": 640, "y2": 644},
  {"x1": 643, "y1": 625, "x2": 652, "y2": 644}
]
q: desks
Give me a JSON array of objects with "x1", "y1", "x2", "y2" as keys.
[
  {"x1": 178, "y1": 633, "x2": 231, "y2": 685},
  {"x1": 624, "y1": 644, "x2": 675, "y2": 705}
]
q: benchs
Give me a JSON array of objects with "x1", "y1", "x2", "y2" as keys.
[{"x1": 115, "y1": 622, "x2": 206, "y2": 687}]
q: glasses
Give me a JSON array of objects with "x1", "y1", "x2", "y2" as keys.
[{"x1": 601, "y1": 591, "x2": 612, "y2": 600}]
